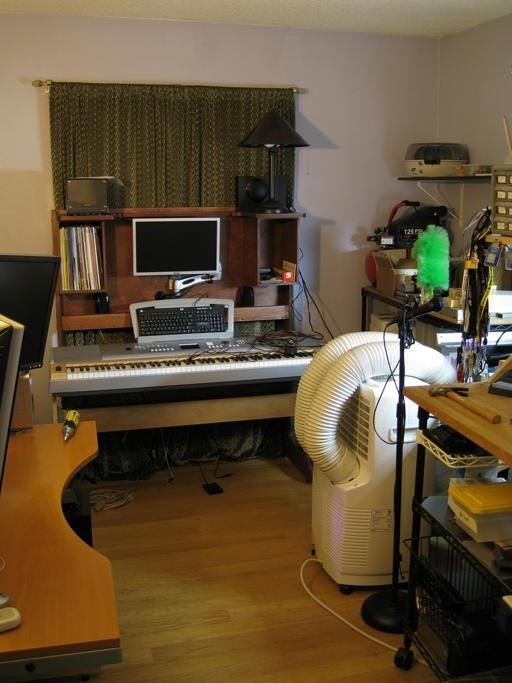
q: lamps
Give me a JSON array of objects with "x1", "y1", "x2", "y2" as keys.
[{"x1": 236, "y1": 102, "x2": 309, "y2": 210}]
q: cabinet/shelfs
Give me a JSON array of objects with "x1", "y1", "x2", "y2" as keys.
[
  {"x1": 394, "y1": 380, "x2": 512, "y2": 682},
  {"x1": 53, "y1": 206, "x2": 299, "y2": 334},
  {"x1": 353, "y1": 280, "x2": 466, "y2": 351}
]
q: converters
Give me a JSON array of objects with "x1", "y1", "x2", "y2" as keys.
[{"x1": 203, "y1": 482, "x2": 223, "y2": 495}]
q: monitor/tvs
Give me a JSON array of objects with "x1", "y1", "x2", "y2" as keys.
[
  {"x1": 0, "y1": 254, "x2": 61, "y2": 431},
  {"x1": 132, "y1": 218, "x2": 222, "y2": 277}
]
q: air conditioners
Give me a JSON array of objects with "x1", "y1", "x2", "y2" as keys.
[{"x1": 292, "y1": 329, "x2": 444, "y2": 594}]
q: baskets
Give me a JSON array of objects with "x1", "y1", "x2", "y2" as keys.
[{"x1": 403, "y1": 532, "x2": 511, "y2": 665}]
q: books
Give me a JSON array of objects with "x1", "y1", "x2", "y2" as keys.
[{"x1": 60, "y1": 226, "x2": 102, "y2": 291}]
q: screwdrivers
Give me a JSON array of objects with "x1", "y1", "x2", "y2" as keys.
[{"x1": 62, "y1": 410, "x2": 80, "y2": 441}]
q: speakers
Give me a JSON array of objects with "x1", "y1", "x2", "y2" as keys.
[
  {"x1": 240, "y1": 287, "x2": 254, "y2": 307},
  {"x1": 236, "y1": 176, "x2": 286, "y2": 211},
  {"x1": 94, "y1": 292, "x2": 109, "y2": 313}
]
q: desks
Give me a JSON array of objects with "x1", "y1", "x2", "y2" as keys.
[
  {"x1": 0, "y1": 416, "x2": 125, "y2": 679},
  {"x1": 49, "y1": 374, "x2": 297, "y2": 437}
]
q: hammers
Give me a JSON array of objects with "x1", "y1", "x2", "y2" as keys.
[{"x1": 429, "y1": 387, "x2": 501, "y2": 424}]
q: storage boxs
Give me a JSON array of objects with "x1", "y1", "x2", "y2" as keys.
[
  {"x1": 371, "y1": 247, "x2": 419, "y2": 297},
  {"x1": 447, "y1": 483, "x2": 511, "y2": 543}
]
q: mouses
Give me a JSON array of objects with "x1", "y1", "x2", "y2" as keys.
[{"x1": 284, "y1": 346, "x2": 297, "y2": 358}]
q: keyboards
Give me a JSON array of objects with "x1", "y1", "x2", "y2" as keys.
[{"x1": 129, "y1": 298, "x2": 235, "y2": 343}]
